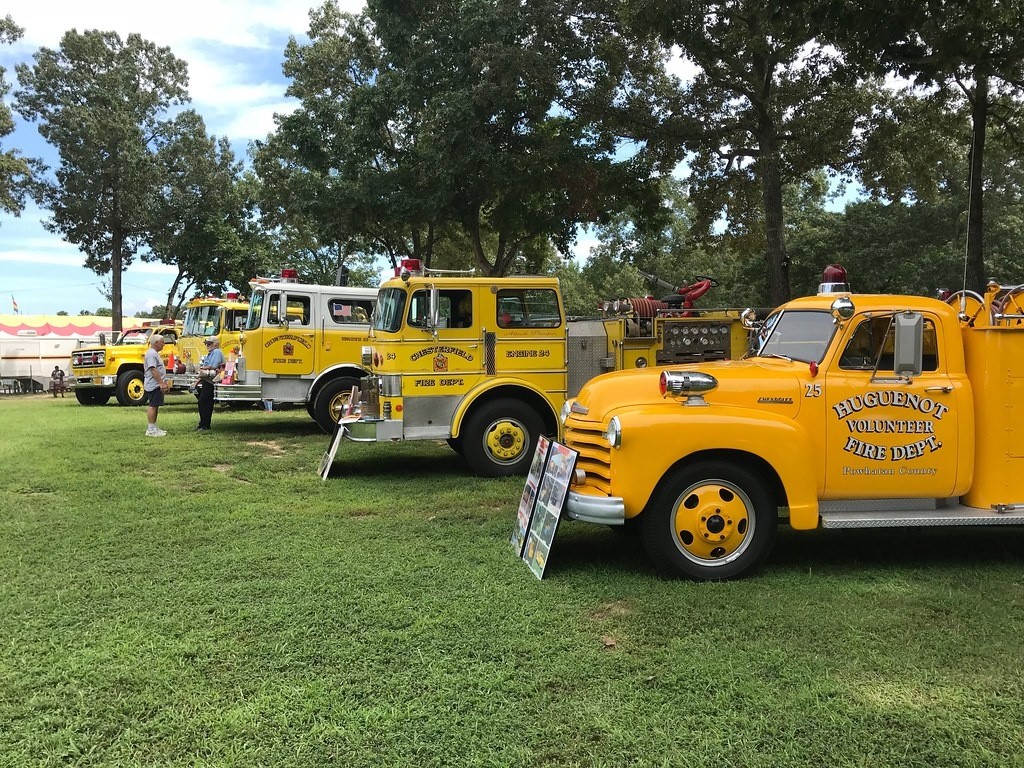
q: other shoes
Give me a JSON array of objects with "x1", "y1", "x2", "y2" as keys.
[
  {"x1": 200, "y1": 427, "x2": 211, "y2": 431},
  {"x1": 194, "y1": 427, "x2": 199, "y2": 430}
]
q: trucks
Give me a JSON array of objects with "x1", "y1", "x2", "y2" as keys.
[{"x1": 560, "y1": 265, "x2": 1023, "y2": 582}]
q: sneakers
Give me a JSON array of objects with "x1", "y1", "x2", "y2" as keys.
[
  {"x1": 157, "y1": 428, "x2": 167, "y2": 433},
  {"x1": 145, "y1": 429, "x2": 166, "y2": 437}
]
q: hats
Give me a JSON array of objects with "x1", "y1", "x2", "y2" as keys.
[{"x1": 206, "y1": 336, "x2": 219, "y2": 343}]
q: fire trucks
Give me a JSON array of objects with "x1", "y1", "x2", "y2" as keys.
[
  {"x1": 337, "y1": 258, "x2": 935, "y2": 477},
  {"x1": 66, "y1": 321, "x2": 214, "y2": 407},
  {"x1": 213, "y1": 268, "x2": 448, "y2": 435},
  {"x1": 163, "y1": 292, "x2": 304, "y2": 411}
]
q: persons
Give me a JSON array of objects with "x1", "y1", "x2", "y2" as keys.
[
  {"x1": 143, "y1": 334, "x2": 168, "y2": 437},
  {"x1": 51, "y1": 366, "x2": 65, "y2": 398},
  {"x1": 189, "y1": 336, "x2": 227, "y2": 430}
]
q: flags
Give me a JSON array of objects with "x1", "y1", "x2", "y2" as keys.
[{"x1": 13, "y1": 301, "x2": 18, "y2": 312}]
